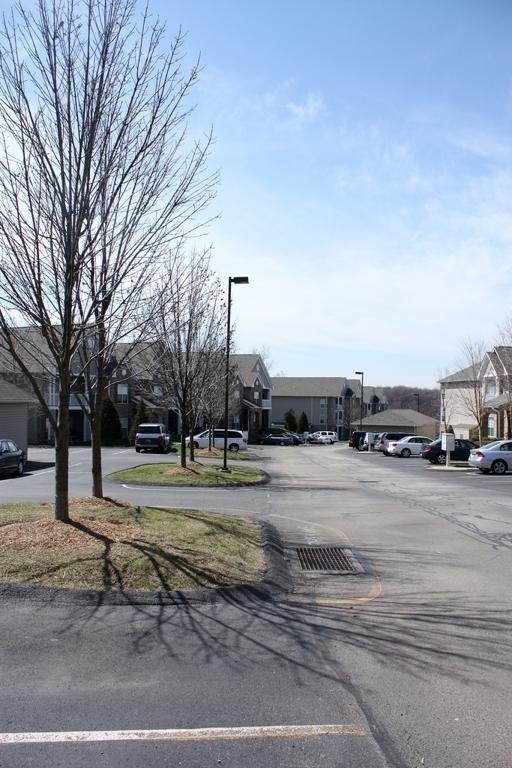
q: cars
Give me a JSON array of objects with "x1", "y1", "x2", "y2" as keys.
[
  {"x1": 0, "y1": 439, "x2": 28, "y2": 476},
  {"x1": 348, "y1": 428, "x2": 512, "y2": 475},
  {"x1": 257, "y1": 429, "x2": 340, "y2": 447}
]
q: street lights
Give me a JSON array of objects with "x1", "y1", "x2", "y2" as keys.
[
  {"x1": 412, "y1": 392, "x2": 419, "y2": 412},
  {"x1": 355, "y1": 371, "x2": 363, "y2": 431},
  {"x1": 219, "y1": 275, "x2": 250, "y2": 470}
]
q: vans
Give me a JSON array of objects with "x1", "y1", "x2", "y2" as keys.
[{"x1": 184, "y1": 427, "x2": 248, "y2": 452}]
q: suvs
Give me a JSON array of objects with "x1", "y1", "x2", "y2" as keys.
[{"x1": 135, "y1": 422, "x2": 172, "y2": 453}]
